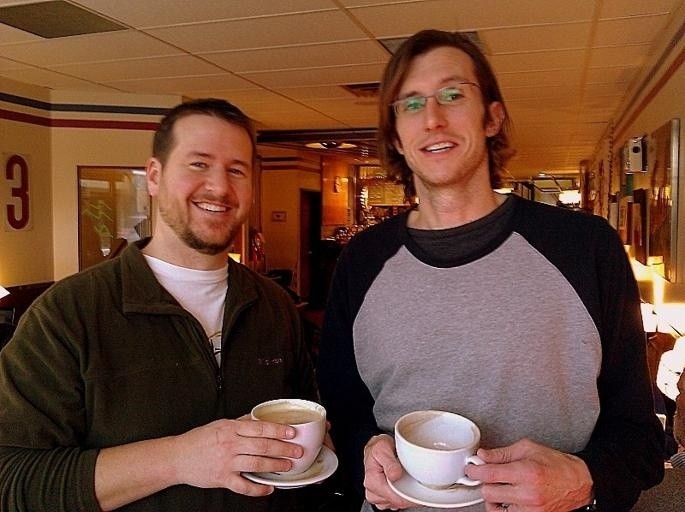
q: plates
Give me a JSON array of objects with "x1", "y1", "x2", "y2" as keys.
[
  {"x1": 385, "y1": 456, "x2": 487, "y2": 508},
  {"x1": 239, "y1": 443, "x2": 339, "y2": 492}
]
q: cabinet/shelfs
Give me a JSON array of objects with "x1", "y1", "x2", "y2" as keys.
[{"x1": 316, "y1": 237, "x2": 340, "y2": 310}]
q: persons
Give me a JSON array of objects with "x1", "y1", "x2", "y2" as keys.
[
  {"x1": 316, "y1": 30, "x2": 666, "y2": 512},
  {"x1": 1, "y1": 99, "x2": 338, "y2": 509}
]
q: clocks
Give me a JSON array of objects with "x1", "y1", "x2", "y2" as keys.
[{"x1": 334, "y1": 174, "x2": 343, "y2": 194}]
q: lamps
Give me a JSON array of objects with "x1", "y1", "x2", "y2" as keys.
[{"x1": 654, "y1": 334, "x2": 685, "y2": 459}]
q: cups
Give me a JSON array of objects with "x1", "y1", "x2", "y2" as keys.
[
  {"x1": 253, "y1": 398, "x2": 330, "y2": 476},
  {"x1": 391, "y1": 410, "x2": 488, "y2": 488}
]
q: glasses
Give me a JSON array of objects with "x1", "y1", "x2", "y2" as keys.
[{"x1": 391, "y1": 82, "x2": 483, "y2": 119}]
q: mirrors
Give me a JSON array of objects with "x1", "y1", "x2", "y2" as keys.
[{"x1": 76, "y1": 163, "x2": 154, "y2": 273}]
